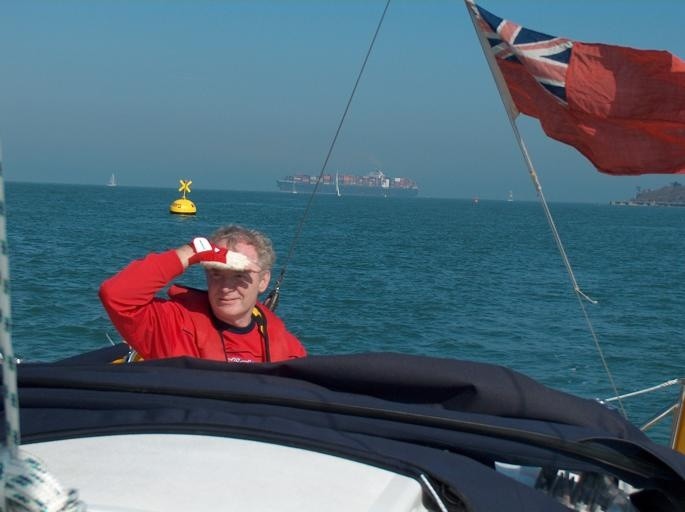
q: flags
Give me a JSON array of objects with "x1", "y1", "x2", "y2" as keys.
[{"x1": 472, "y1": 5, "x2": 685, "y2": 176}]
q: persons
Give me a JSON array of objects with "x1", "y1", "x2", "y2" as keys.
[{"x1": 98, "y1": 225, "x2": 305, "y2": 364}]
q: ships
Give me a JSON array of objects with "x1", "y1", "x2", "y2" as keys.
[{"x1": 276, "y1": 166, "x2": 420, "y2": 199}]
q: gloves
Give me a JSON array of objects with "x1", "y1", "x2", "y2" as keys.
[{"x1": 182, "y1": 235, "x2": 249, "y2": 272}]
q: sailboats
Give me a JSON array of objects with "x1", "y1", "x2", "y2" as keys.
[{"x1": 107, "y1": 173, "x2": 118, "y2": 186}]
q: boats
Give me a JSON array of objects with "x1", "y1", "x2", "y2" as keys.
[{"x1": 0, "y1": 344, "x2": 685, "y2": 512}]
